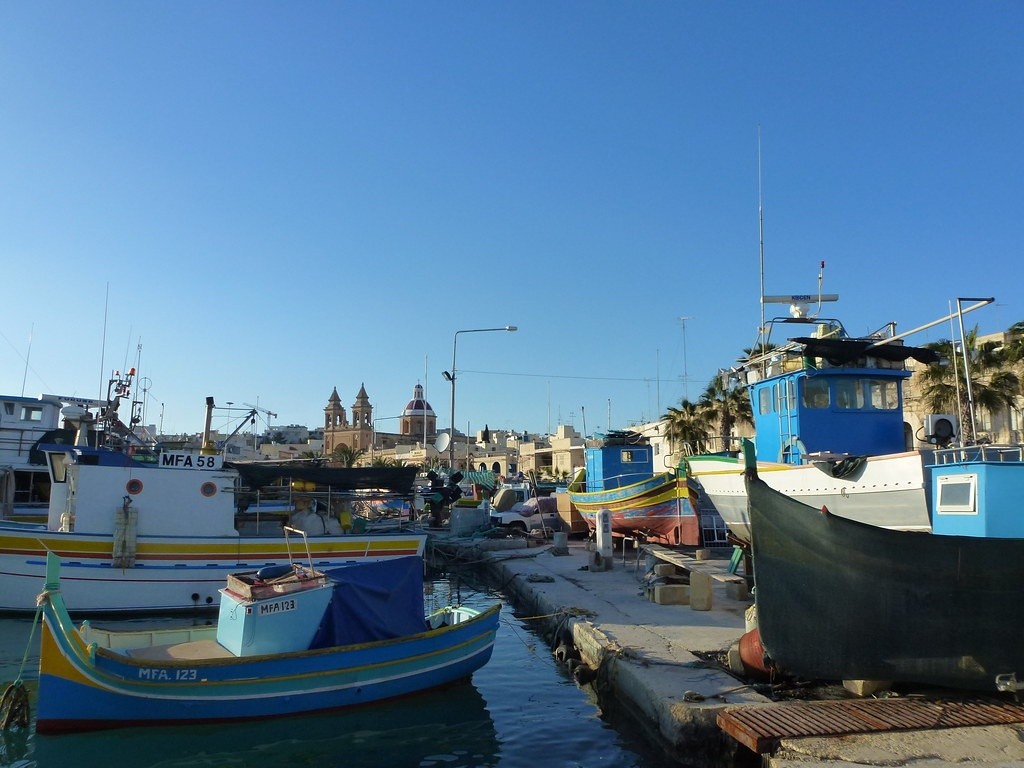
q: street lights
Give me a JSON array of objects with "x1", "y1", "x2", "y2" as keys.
[{"x1": 441, "y1": 325, "x2": 518, "y2": 479}]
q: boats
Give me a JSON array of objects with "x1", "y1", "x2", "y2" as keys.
[
  {"x1": 0, "y1": 278, "x2": 428, "y2": 621},
  {"x1": 34, "y1": 526, "x2": 503, "y2": 720},
  {"x1": 741, "y1": 439, "x2": 1024, "y2": 697},
  {"x1": 564, "y1": 429, "x2": 699, "y2": 546},
  {"x1": 686, "y1": 260, "x2": 1024, "y2": 540}
]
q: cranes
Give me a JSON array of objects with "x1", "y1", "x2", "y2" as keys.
[{"x1": 242, "y1": 403, "x2": 277, "y2": 431}]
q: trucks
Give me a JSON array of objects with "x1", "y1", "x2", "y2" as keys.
[{"x1": 492, "y1": 485, "x2": 566, "y2": 513}]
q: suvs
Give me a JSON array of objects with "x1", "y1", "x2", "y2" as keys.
[{"x1": 490, "y1": 496, "x2": 565, "y2": 534}]
